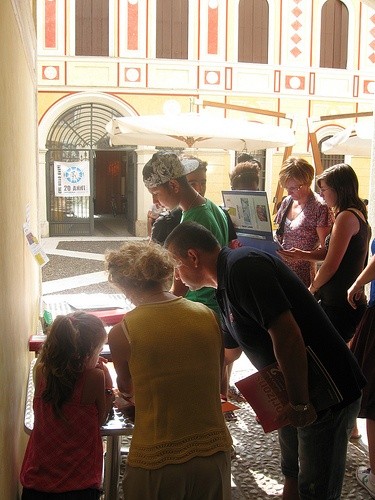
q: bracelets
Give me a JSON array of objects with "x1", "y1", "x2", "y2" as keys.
[
  {"x1": 289, "y1": 403, "x2": 311, "y2": 413},
  {"x1": 311, "y1": 282, "x2": 317, "y2": 290}
]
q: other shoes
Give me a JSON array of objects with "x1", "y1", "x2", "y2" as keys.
[
  {"x1": 228, "y1": 385, "x2": 243, "y2": 400},
  {"x1": 220, "y1": 398, "x2": 237, "y2": 421}
]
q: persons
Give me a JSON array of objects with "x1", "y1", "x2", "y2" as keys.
[
  {"x1": 346, "y1": 236, "x2": 375, "y2": 498},
  {"x1": 273, "y1": 156, "x2": 336, "y2": 287},
  {"x1": 19, "y1": 312, "x2": 114, "y2": 500},
  {"x1": 105, "y1": 237, "x2": 233, "y2": 500},
  {"x1": 229, "y1": 160, "x2": 263, "y2": 190},
  {"x1": 308, "y1": 163, "x2": 371, "y2": 440},
  {"x1": 164, "y1": 220, "x2": 367, "y2": 500},
  {"x1": 150, "y1": 157, "x2": 240, "y2": 296}
]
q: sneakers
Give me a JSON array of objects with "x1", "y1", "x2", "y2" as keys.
[{"x1": 356, "y1": 465, "x2": 375, "y2": 499}]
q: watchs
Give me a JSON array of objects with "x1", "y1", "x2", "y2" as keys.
[{"x1": 104, "y1": 386, "x2": 114, "y2": 399}]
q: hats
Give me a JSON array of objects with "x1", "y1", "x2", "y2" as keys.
[{"x1": 142, "y1": 151, "x2": 200, "y2": 189}]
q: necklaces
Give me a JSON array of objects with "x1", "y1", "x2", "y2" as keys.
[
  {"x1": 145, "y1": 290, "x2": 172, "y2": 301},
  {"x1": 187, "y1": 191, "x2": 200, "y2": 210}
]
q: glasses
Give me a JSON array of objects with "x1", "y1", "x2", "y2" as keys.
[{"x1": 284, "y1": 183, "x2": 303, "y2": 193}]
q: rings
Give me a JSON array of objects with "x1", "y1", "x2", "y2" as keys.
[{"x1": 285, "y1": 260, "x2": 287, "y2": 263}]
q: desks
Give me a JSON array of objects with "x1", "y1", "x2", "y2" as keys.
[{"x1": 24, "y1": 358, "x2": 135, "y2": 500}]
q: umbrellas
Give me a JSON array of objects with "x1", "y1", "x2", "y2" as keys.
[
  {"x1": 320, "y1": 119, "x2": 375, "y2": 158},
  {"x1": 105, "y1": 113, "x2": 297, "y2": 153}
]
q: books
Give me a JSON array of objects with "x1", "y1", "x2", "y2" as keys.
[{"x1": 221, "y1": 190, "x2": 290, "y2": 263}]
